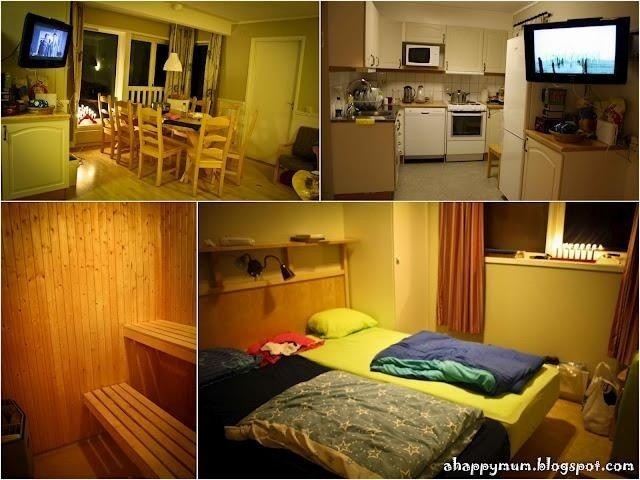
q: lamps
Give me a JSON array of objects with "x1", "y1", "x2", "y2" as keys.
[
  {"x1": 162, "y1": 3, "x2": 183, "y2": 73},
  {"x1": 240, "y1": 252, "x2": 295, "y2": 282}
]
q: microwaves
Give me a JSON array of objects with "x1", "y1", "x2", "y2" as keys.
[{"x1": 405, "y1": 44, "x2": 440, "y2": 68}]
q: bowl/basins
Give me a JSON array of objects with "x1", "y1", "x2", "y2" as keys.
[
  {"x1": 151, "y1": 102, "x2": 171, "y2": 114},
  {"x1": 549, "y1": 128, "x2": 583, "y2": 143}
]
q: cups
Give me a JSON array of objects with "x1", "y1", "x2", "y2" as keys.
[
  {"x1": 384, "y1": 105, "x2": 388, "y2": 110},
  {"x1": 394, "y1": 97, "x2": 401, "y2": 105},
  {"x1": 388, "y1": 106, "x2": 392, "y2": 110},
  {"x1": 386, "y1": 96, "x2": 393, "y2": 104},
  {"x1": 383, "y1": 97, "x2": 389, "y2": 106}
]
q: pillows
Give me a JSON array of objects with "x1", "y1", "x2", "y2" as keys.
[
  {"x1": 199, "y1": 347, "x2": 255, "y2": 385},
  {"x1": 307, "y1": 307, "x2": 378, "y2": 339}
]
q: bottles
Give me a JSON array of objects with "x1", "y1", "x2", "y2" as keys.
[
  {"x1": 26, "y1": 75, "x2": 36, "y2": 103},
  {"x1": 334, "y1": 96, "x2": 343, "y2": 119},
  {"x1": 182, "y1": 103, "x2": 188, "y2": 118}
]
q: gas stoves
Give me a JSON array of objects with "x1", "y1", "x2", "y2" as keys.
[{"x1": 444, "y1": 98, "x2": 485, "y2": 114}]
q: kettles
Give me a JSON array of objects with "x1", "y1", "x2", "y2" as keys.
[{"x1": 401, "y1": 85, "x2": 418, "y2": 103}]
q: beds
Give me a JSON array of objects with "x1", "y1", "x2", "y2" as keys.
[{"x1": 199, "y1": 326, "x2": 560, "y2": 479}]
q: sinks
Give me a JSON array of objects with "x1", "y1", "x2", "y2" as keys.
[{"x1": 353, "y1": 110, "x2": 391, "y2": 117}]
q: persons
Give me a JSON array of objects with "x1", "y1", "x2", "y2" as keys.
[
  {"x1": 38, "y1": 32, "x2": 59, "y2": 58},
  {"x1": 537, "y1": 57, "x2": 588, "y2": 74}
]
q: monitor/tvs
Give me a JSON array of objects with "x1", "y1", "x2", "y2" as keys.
[
  {"x1": 17, "y1": 10, "x2": 73, "y2": 68},
  {"x1": 522, "y1": 15, "x2": 631, "y2": 86}
]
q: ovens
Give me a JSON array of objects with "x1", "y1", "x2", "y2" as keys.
[{"x1": 446, "y1": 111, "x2": 487, "y2": 161}]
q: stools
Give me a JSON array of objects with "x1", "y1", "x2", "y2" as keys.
[{"x1": 487, "y1": 143, "x2": 502, "y2": 189}]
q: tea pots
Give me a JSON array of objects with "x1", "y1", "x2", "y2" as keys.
[{"x1": 415, "y1": 84, "x2": 427, "y2": 104}]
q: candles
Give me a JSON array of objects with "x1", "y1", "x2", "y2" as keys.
[{"x1": 551, "y1": 244, "x2": 605, "y2": 262}]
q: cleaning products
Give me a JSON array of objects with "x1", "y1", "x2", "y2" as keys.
[{"x1": 335, "y1": 96, "x2": 342, "y2": 120}]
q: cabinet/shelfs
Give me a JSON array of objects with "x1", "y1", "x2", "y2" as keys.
[
  {"x1": 484, "y1": 28, "x2": 514, "y2": 74},
  {"x1": 405, "y1": 108, "x2": 446, "y2": 156},
  {"x1": 521, "y1": 129, "x2": 639, "y2": 200},
  {"x1": 1, "y1": 119, "x2": 69, "y2": 200},
  {"x1": 444, "y1": 25, "x2": 483, "y2": 72},
  {"x1": 404, "y1": 22, "x2": 447, "y2": 45},
  {"x1": 327, "y1": 1, "x2": 379, "y2": 68},
  {"x1": 380, "y1": 14, "x2": 404, "y2": 69}
]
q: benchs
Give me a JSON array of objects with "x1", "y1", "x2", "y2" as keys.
[{"x1": 83, "y1": 383, "x2": 196, "y2": 478}]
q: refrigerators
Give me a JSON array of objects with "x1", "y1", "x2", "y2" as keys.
[{"x1": 497, "y1": 34, "x2": 589, "y2": 201}]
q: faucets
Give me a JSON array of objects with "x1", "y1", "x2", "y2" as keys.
[{"x1": 346, "y1": 78, "x2": 372, "y2": 118}]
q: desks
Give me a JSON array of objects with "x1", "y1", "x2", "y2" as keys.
[{"x1": 123, "y1": 319, "x2": 196, "y2": 365}]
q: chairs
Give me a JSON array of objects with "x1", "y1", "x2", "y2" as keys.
[
  {"x1": 97, "y1": 92, "x2": 258, "y2": 199},
  {"x1": 273, "y1": 125, "x2": 319, "y2": 186}
]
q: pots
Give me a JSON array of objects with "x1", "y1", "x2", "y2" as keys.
[{"x1": 446, "y1": 89, "x2": 471, "y2": 105}]
